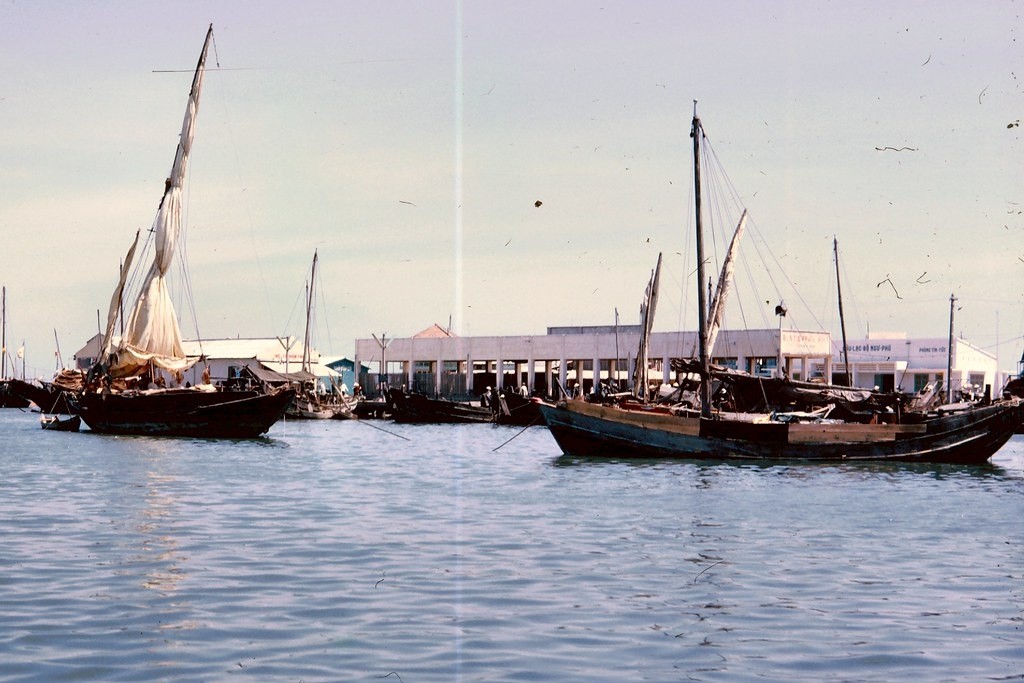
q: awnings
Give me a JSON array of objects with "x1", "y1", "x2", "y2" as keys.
[{"x1": 261, "y1": 361, "x2": 342, "y2": 377}]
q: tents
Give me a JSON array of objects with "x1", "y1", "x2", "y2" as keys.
[{"x1": 238, "y1": 363, "x2": 320, "y2": 385}]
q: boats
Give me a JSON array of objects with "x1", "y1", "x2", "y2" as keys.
[{"x1": 357, "y1": 383, "x2": 545, "y2": 426}]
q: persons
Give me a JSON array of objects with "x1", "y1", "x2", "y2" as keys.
[
  {"x1": 521, "y1": 382, "x2": 529, "y2": 397},
  {"x1": 934, "y1": 391, "x2": 946, "y2": 408},
  {"x1": 573, "y1": 382, "x2": 579, "y2": 398}
]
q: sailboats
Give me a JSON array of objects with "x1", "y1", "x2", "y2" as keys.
[
  {"x1": 532, "y1": 96, "x2": 1024, "y2": 464},
  {"x1": 267, "y1": 244, "x2": 361, "y2": 419},
  {"x1": 0, "y1": 284, "x2": 83, "y2": 419},
  {"x1": 35, "y1": 21, "x2": 295, "y2": 441}
]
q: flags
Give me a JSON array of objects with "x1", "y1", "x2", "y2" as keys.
[{"x1": 17, "y1": 346, "x2": 25, "y2": 358}]
